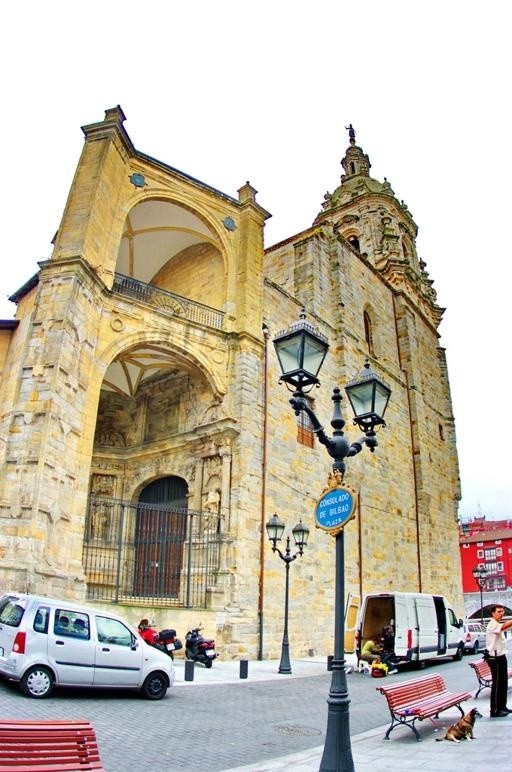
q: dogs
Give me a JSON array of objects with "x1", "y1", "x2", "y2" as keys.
[{"x1": 435, "y1": 707, "x2": 483, "y2": 743}]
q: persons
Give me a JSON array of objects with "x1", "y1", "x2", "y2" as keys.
[
  {"x1": 380, "y1": 625, "x2": 399, "y2": 674},
  {"x1": 361, "y1": 636, "x2": 383, "y2": 664},
  {"x1": 484, "y1": 605, "x2": 512, "y2": 717}
]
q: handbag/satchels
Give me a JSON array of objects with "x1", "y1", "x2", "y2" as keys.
[{"x1": 370, "y1": 663, "x2": 389, "y2": 678}]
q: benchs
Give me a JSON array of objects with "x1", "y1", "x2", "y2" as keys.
[
  {"x1": 1, "y1": 719, "x2": 103, "y2": 772},
  {"x1": 469, "y1": 659, "x2": 512, "y2": 698},
  {"x1": 375, "y1": 673, "x2": 474, "y2": 741}
]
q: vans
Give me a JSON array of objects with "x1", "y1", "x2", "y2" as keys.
[{"x1": 344, "y1": 592, "x2": 465, "y2": 670}]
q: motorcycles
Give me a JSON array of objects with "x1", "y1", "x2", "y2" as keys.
[{"x1": 138, "y1": 619, "x2": 219, "y2": 668}]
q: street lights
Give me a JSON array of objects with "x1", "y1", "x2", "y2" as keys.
[
  {"x1": 472, "y1": 566, "x2": 488, "y2": 623},
  {"x1": 272, "y1": 308, "x2": 391, "y2": 772},
  {"x1": 266, "y1": 512, "x2": 309, "y2": 674}
]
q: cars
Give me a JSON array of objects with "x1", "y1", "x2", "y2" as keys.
[
  {"x1": 0, "y1": 593, "x2": 175, "y2": 700},
  {"x1": 464, "y1": 623, "x2": 486, "y2": 654}
]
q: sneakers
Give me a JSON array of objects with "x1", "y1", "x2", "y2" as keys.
[
  {"x1": 388, "y1": 669, "x2": 399, "y2": 675},
  {"x1": 490, "y1": 705, "x2": 512, "y2": 717}
]
